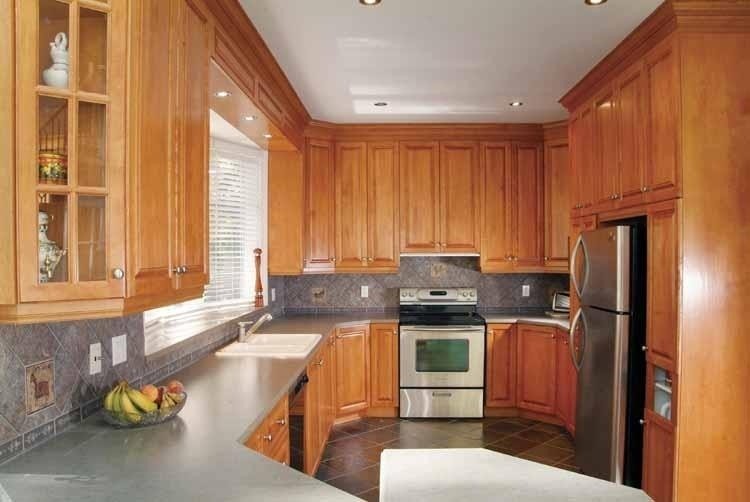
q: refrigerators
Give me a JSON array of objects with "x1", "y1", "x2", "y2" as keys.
[{"x1": 571, "y1": 222, "x2": 644, "y2": 490}]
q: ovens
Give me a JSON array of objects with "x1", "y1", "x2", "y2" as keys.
[{"x1": 399, "y1": 323, "x2": 486, "y2": 420}]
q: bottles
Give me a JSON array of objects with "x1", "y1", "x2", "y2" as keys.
[
  {"x1": 252, "y1": 248, "x2": 264, "y2": 306},
  {"x1": 41, "y1": 30, "x2": 70, "y2": 89}
]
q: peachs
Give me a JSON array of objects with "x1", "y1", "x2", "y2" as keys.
[
  {"x1": 156, "y1": 386, "x2": 167, "y2": 404},
  {"x1": 168, "y1": 380, "x2": 184, "y2": 392},
  {"x1": 140, "y1": 384, "x2": 159, "y2": 401}
]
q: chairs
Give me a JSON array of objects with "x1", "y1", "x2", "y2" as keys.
[{"x1": 371, "y1": 443, "x2": 655, "y2": 502}]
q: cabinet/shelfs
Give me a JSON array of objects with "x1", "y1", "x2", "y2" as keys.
[
  {"x1": 335, "y1": 122, "x2": 399, "y2": 274},
  {"x1": 239, "y1": 337, "x2": 331, "y2": 476},
  {"x1": 596, "y1": 57, "x2": 648, "y2": 223},
  {"x1": 399, "y1": 122, "x2": 481, "y2": 257},
  {"x1": 125, "y1": 0, "x2": 213, "y2": 315},
  {"x1": 265, "y1": 119, "x2": 336, "y2": 277},
  {"x1": 334, "y1": 325, "x2": 369, "y2": 416},
  {"x1": 0, "y1": 1, "x2": 125, "y2": 324},
  {"x1": 488, "y1": 324, "x2": 516, "y2": 403},
  {"x1": 482, "y1": 122, "x2": 542, "y2": 276},
  {"x1": 556, "y1": 330, "x2": 580, "y2": 431},
  {"x1": 370, "y1": 325, "x2": 399, "y2": 409},
  {"x1": 542, "y1": 119, "x2": 570, "y2": 276},
  {"x1": 517, "y1": 325, "x2": 557, "y2": 418},
  {"x1": 568, "y1": 212, "x2": 621, "y2": 331},
  {"x1": 646, "y1": 14, "x2": 750, "y2": 202},
  {"x1": 555, "y1": 82, "x2": 596, "y2": 221},
  {"x1": 638, "y1": 195, "x2": 748, "y2": 502}
]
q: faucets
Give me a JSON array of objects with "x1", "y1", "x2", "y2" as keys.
[{"x1": 238, "y1": 313, "x2": 272, "y2": 343}]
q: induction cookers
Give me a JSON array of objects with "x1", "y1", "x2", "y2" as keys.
[{"x1": 398, "y1": 312, "x2": 484, "y2": 326}]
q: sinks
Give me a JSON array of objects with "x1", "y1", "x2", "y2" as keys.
[
  {"x1": 232, "y1": 333, "x2": 323, "y2": 345},
  {"x1": 215, "y1": 344, "x2": 311, "y2": 359}
]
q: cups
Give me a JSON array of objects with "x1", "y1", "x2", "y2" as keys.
[{"x1": 39, "y1": 147, "x2": 68, "y2": 186}]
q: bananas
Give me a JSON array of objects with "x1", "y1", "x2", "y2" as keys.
[
  {"x1": 160, "y1": 392, "x2": 185, "y2": 409},
  {"x1": 104, "y1": 379, "x2": 157, "y2": 424}
]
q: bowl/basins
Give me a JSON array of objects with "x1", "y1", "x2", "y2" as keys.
[{"x1": 98, "y1": 391, "x2": 188, "y2": 428}]
